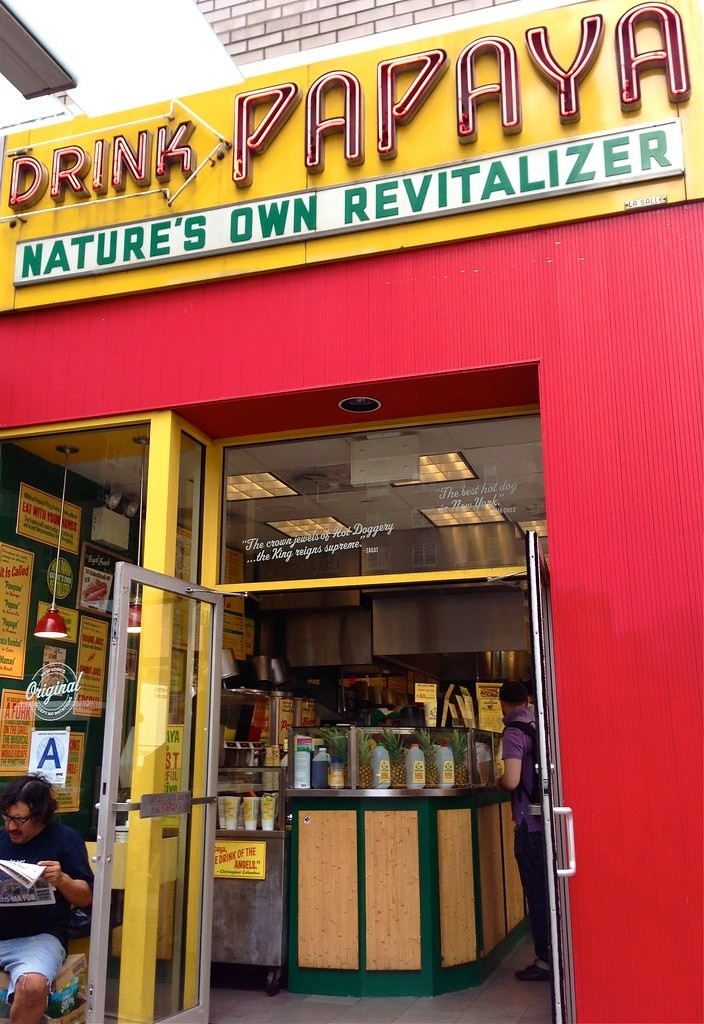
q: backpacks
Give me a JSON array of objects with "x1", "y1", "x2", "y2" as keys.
[{"x1": 501, "y1": 721, "x2": 542, "y2": 804}]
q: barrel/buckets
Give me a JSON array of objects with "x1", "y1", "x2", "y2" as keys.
[
  {"x1": 354, "y1": 681, "x2": 396, "y2": 707},
  {"x1": 249, "y1": 655, "x2": 272, "y2": 684},
  {"x1": 221, "y1": 648, "x2": 240, "y2": 680},
  {"x1": 271, "y1": 658, "x2": 290, "y2": 687}
]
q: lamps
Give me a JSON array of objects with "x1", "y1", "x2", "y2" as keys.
[
  {"x1": 127, "y1": 434, "x2": 151, "y2": 634},
  {"x1": 91, "y1": 491, "x2": 139, "y2": 551},
  {"x1": 34, "y1": 445, "x2": 80, "y2": 639}
]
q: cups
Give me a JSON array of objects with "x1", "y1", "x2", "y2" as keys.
[
  {"x1": 260, "y1": 797, "x2": 275, "y2": 831},
  {"x1": 223, "y1": 797, "x2": 240, "y2": 830},
  {"x1": 243, "y1": 797, "x2": 259, "y2": 830}
]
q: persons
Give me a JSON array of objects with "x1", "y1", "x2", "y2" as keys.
[
  {"x1": 496, "y1": 679, "x2": 556, "y2": 984},
  {"x1": 1, "y1": 775, "x2": 94, "y2": 1024},
  {"x1": 370, "y1": 708, "x2": 400, "y2": 726}
]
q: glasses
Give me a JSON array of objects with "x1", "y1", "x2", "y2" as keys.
[{"x1": 1, "y1": 814, "x2": 32, "y2": 825}]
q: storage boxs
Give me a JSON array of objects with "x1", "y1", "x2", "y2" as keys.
[{"x1": 0, "y1": 953, "x2": 88, "y2": 1024}]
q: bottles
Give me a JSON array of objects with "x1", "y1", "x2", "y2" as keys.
[
  {"x1": 370, "y1": 743, "x2": 391, "y2": 789},
  {"x1": 435, "y1": 744, "x2": 455, "y2": 788},
  {"x1": 312, "y1": 748, "x2": 329, "y2": 789},
  {"x1": 328, "y1": 758, "x2": 345, "y2": 789},
  {"x1": 405, "y1": 744, "x2": 426, "y2": 789}
]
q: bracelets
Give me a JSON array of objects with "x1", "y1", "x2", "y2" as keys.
[{"x1": 499, "y1": 776, "x2": 504, "y2": 789}]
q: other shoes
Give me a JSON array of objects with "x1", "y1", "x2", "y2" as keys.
[{"x1": 515, "y1": 964, "x2": 552, "y2": 980}]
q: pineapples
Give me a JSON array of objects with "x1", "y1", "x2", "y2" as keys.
[{"x1": 319, "y1": 727, "x2": 470, "y2": 789}]
